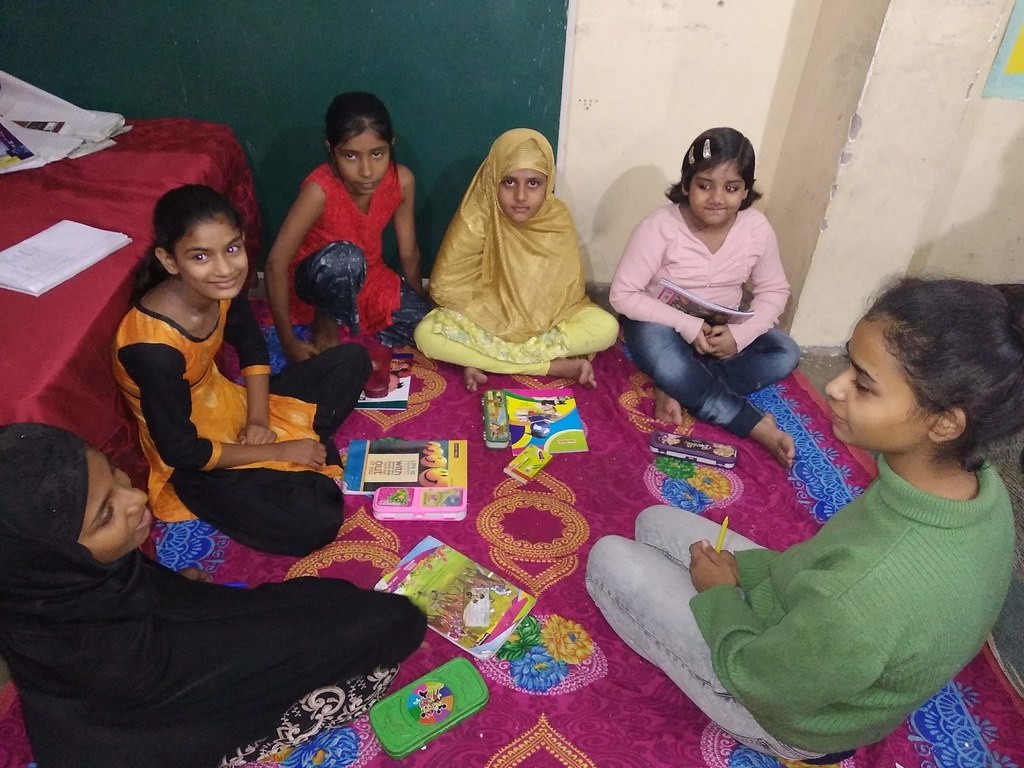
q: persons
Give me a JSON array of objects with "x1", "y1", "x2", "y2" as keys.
[
  {"x1": 585, "y1": 279, "x2": 1024, "y2": 765},
  {"x1": 609, "y1": 127, "x2": 801, "y2": 469},
  {"x1": 263, "y1": 92, "x2": 430, "y2": 365},
  {"x1": 110, "y1": 185, "x2": 345, "y2": 558},
  {"x1": 413, "y1": 128, "x2": 620, "y2": 392},
  {"x1": 0, "y1": 422, "x2": 429, "y2": 768}
]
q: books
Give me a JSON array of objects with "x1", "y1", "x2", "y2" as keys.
[
  {"x1": 374, "y1": 535, "x2": 539, "y2": 662},
  {"x1": 502, "y1": 388, "x2": 589, "y2": 457},
  {"x1": 653, "y1": 277, "x2": 755, "y2": 324},
  {"x1": 341, "y1": 439, "x2": 468, "y2": 495},
  {"x1": 353, "y1": 353, "x2": 414, "y2": 411}
]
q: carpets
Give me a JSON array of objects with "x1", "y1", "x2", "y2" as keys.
[{"x1": 0, "y1": 321, "x2": 1024, "y2": 768}]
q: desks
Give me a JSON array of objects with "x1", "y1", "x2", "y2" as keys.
[{"x1": 0, "y1": 121, "x2": 263, "y2": 476}]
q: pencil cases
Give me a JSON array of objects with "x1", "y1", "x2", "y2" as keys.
[
  {"x1": 649, "y1": 430, "x2": 738, "y2": 469},
  {"x1": 372, "y1": 486, "x2": 467, "y2": 523},
  {"x1": 362, "y1": 344, "x2": 393, "y2": 398},
  {"x1": 482, "y1": 389, "x2": 511, "y2": 449},
  {"x1": 367, "y1": 654, "x2": 491, "y2": 759}
]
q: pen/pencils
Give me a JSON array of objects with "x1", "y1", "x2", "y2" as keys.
[{"x1": 715, "y1": 516, "x2": 729, "y2": 553}]
